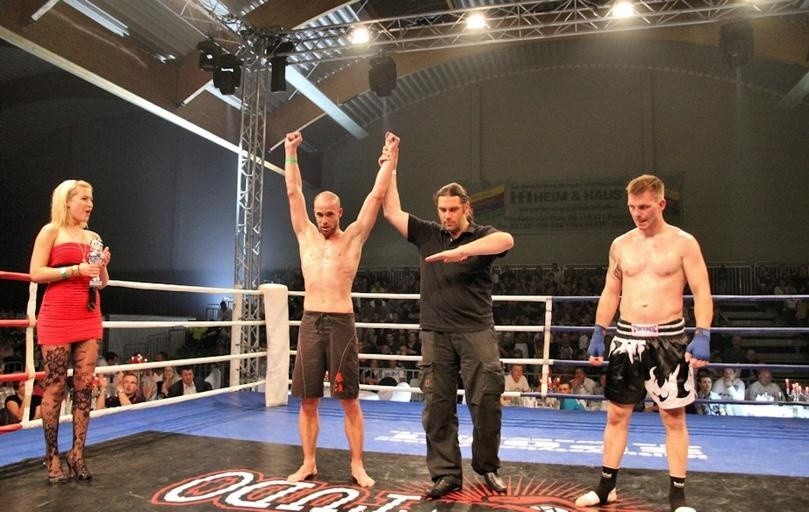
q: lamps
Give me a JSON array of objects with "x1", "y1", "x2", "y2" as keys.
[
  {"x1": 211, "y1": 53, "x2": 242, "y2": 95},
  {"x1": 367, "y1": 39, "x2": 400, "y2": 99},
  {"x1": 264, "y1": 41, "x2": 296, "y2": 94},
  {"x1": 195, "y1": 41, "x2": 221, "y2": 71},
  {"x1": 717, "y1": 14, "x2": 755, "y2": 69}
]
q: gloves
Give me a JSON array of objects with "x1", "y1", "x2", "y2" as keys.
[
  {"x1": 687, "y1": 328, "x2": 710, "y2": 361},
  {"x1": 588, "y1": 326, "x2": 607, "y2": 357}
]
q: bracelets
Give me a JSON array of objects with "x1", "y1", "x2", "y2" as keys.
[
  {"x1": 59, "y1": 266, "x2": 68, "y2": 280},
  {"x1": 71, "y1": 264, "x2": 80, "y2": 277},
  {"x1": 284, "y1": 158, "x2": 297, "y2": 164}
]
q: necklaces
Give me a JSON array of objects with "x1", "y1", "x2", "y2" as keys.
[{"x1": 66, "y1": 227, "x2": 87, "y2": 262}]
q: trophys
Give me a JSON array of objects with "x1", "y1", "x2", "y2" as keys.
[{"x1": 88, "y1": 238, "x2": 104, "y2": 286}]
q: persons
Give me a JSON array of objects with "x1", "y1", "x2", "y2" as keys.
[
  {"x1": 380, "y1": 345, "x2": 391, "y2": 355},
  {"x1": 571, "y1": 368, "x2": 595, "y2": 394},
  {"x1": 558, "y1": 381, "x2": 584, "y2": 411},
  {"x1": 408, "y1": 299, "x2": 420, "y2": 323},
  {"x1": 739, "y1": 349, "x2": 758, "y2": 377},
  {"x1": 519, "y1": 265, "x2": 530, "y2": 290},
  {"x1": 575, "y1": 174, "x2": 714, "y2": 512},
  {"x1": 407, "y1": 333, "x2": 421, "y2": 356},
  {"x1": 5, "y1": 381, "x2": 42, "y2": 425},
  {"x1": 593, "y1": 371, "x2": 608, "y2": 395},
  {"x1": 501, "y1": 332, "x2": 513, "y2": 356},
  {"x1": 399, "y1": 345, "x2": 407, "y2": 355},
  {"x1": 745, "y1": 369, "x2": 786, "y2": 401},
  {"x1": 723, "y1": 335, "x2": 745, "y2": 363},
  {"x1": 96, "y1": 370, "x2": 142, "y2": 412},
  {"x1": 530, "y1": 266, "x2": 544, "y2": 293},
  {"x1": 694, "y1": 376, "x2": 725, "y2": 417},
  {"x1": 388, "y1": 360, "x2": 404, "y2": 382},
  {"x1": 360, "y1": 299, "x2": 375, "y2": 314},
  {"x1": 712, "y1": 368, "x2": 745, "y2": 401},
  {"x1": 167, "y1": 366, "x2": 212, "y2": 397},
  {"x1": 544, "y1": 273, "x2": 557, "y2": 288},
  {"x1": 29, "y1": 179, "x2": 111, "y2": 484},
  {"x1": 402, "y1": 267, "x2": 415, "y2": 285},
  {"x1": 371, "y1": 282, "x2": 384, "y2": 293},
  {"x1": 698, "y1": 368, "x2": 709, "y2": 377},
  {"x1": 386, "y1": 333, "x2": 400, "y2": 354},
  {"x1": 377, "y1": 147, "x2": 514, "y2": 500},
  {"x1": 361, "y1": 360, "x2": 382, "y2": 385},
  {"x1": 217, "y1": 301, "x2": 232, "y2": 321},
  {"x1": 794, "y1": 264, "x2": 807, "y2": 289},
  {"x1": 285, "y1": 132, "x2": 400, "y2": 488},
  {"x1": 785, "y1": 282, "x2": 799, "y2": 310},
  {"x1": 98, "y1": 351, "x2": 117, "y2": 367},
  {"x1": 154, "y1": 351, "x2": 168, "y2": 362},
  {"x1": 546, "y1": 263, "x2": 561, "y2": 278},
  {"x1": 774, "y1": 280, "x2": 785, "y2": 295},
  {"x1": 504, "y1": 364, "x2": 529, "y2": 392},
  {"x1": 138, "y1": 374, "x2": 158, "y2": 401},
  {"x1": 358, "y1": 341, "x2": 368, "y2": 367},
  {"x1": 156, "y1": 367, "x2": 178, "y2": 398}
]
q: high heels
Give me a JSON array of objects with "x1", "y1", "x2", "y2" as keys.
[
  {"x1": 65, "y1": 455, "x2": 92, "y2": 481},
  {"x1": 48, "y1": 472, "x2": 66, "y2": 484}
]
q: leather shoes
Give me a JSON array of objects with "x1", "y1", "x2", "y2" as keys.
[
  {"x1": 429, "y1": 477, "x2": 461, "y2": 496},
  {"x1": 484, "y1": 471, "x2": 508, "y2": 491}
]
